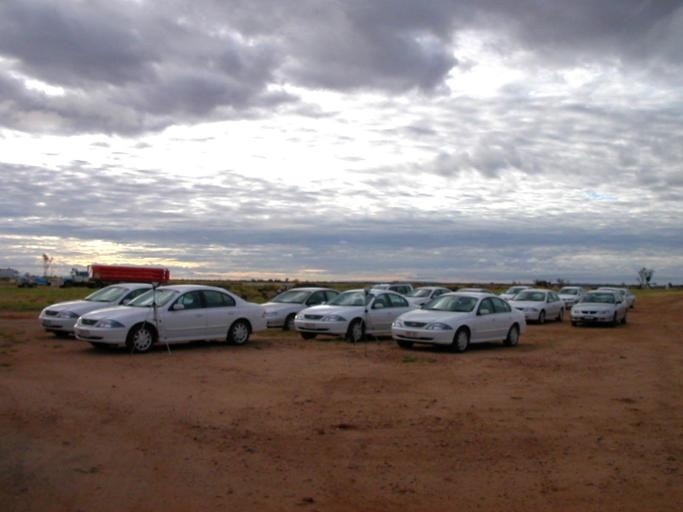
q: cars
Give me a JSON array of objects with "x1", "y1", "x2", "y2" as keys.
[
  {"x1": 73, "y1": 284, "x2": 267, "y2": 353},
  {"x1": 293, "y1": 287, "x2": 422, "y2": 342},
  {"x1": 37, "y1": 282, "x2": 160, "y2": 337},
  {"x1": 390, "y1": 290, "x2": 526, "y2": 353},
  {"x1": 260, "y1": 287, "x2": 340, "y2": 331},
  {"x1": 371, "y1": 281, "x2": 636, "y2": 306}
]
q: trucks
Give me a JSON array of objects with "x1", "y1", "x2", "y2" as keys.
[{"x1": 71, "y1": 262, "x2": 169, "y2": 285}]
